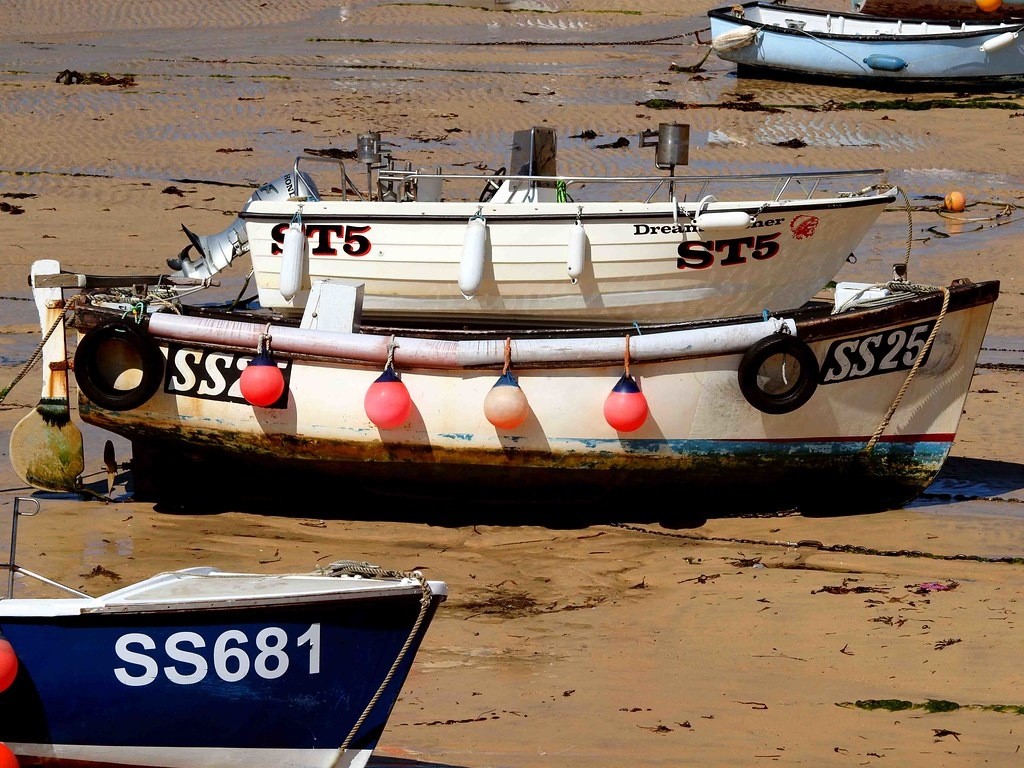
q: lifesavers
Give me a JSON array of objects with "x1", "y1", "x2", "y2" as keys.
[
  {"x1": 738, "y1": 332, "x2": 822, "y2": 417},
  {"x1": 73, "y1": 321, "x2": 164, "y2": 415}
]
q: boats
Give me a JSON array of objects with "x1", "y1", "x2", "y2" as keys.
[
  {"x1": 0, "y1": 497, "x2": 448, "y2": 767},
  {"x1": 9, "y1": 120, "x2": 1001, "y2": 512},
  {"x1": 707, "y1": 1, "x2": 1023, "y2": 80}
]
